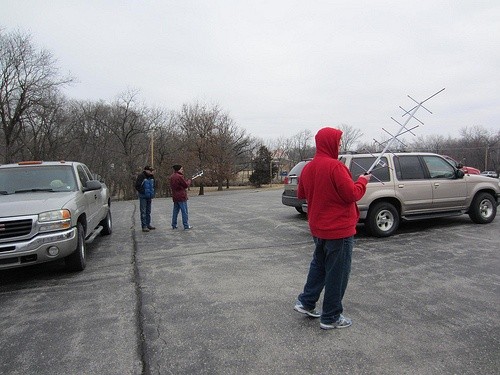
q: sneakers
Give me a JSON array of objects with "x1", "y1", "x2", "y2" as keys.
[
  {"x1": 293, "y1": 299, "x2": 321, "y2": 317},
  {"x1": 320, "y1": 313, "x2": 352, "y2": 330}
]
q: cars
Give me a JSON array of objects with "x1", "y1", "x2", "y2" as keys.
[{"x1": 481, "y1": 171, "x2": 498, "y2": 178}]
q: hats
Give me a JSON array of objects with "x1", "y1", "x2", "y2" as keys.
[
  {"x1": 173, "y1": 164, "x2": 182, "y2": 172},
  {"x1": 144, "y1": 165, "x2": 155, "y2": 171}
]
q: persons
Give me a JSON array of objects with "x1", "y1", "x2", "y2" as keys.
[
  {"x1": 168, "y1": 164, "x2": 193, "y2": 231},
  {"x1": 135, "y1": 166, "x2": 156, "y2": 233},
  {"x1": 292, "y1": 127, "x2": 372, "y2": 329}
]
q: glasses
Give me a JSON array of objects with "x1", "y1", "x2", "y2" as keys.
[{"x1": 338, "y1": 144, "x2": 344, "y2": 148}]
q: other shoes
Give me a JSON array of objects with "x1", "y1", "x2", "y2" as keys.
[
  {"x1": 147, "y1": 225, "x2": 156, "y2": 230},
  {"x1": 183, "y1": 226, "x2": 193, "y2": 231},
  {"x1": 142, "y1": 227, "x2": 149, "y2": 232},
  {"x1": 173, "y1": 225, "x2": 178, "y2": 229}
]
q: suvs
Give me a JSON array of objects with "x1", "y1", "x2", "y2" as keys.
[
  {"x1": 0, "y1": 161, "x2": 112, "y2": 272},
  {"x1": 282, "y1": 158, "x2": 313, "y2": 215},
  {"x1": 301, "y1": 152, "x2": 500, "y2": 238},
  {"x1": 439, "y1": 154, "x2": 481, "y2": 175}
]
